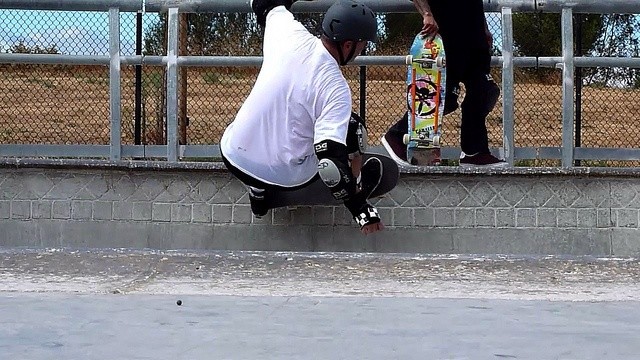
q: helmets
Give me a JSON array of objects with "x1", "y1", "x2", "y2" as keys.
[{"x1": 322, "y1": 1, "x2": 379, "y2": 43}]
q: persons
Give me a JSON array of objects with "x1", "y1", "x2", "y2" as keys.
[
  {"x1": 218, "y1": 0, "x2": 400, "y2": 238},
  {"x1": 380, "y1": 0, "x2": 508, "y2": 168}
]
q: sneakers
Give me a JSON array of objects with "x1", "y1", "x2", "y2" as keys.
[
  {"x1": 357, "y1": 156, "x2": 383, "y2": 200},
  {"x1": 381, "y1": 128, "x2": 420, "y2": 168},
  {"x1": 248, "y1": 194, "x2": 270, "y2": 216},
  {"x1": 458, "y1": 148, "x2": 509, "y2": 168}
]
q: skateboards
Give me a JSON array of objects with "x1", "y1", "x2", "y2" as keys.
[
  {"x1": 403, "y1": 30, "x2": 447, "y2": 166},
  {"x1": 271, "y1": 153, "x2": 399, "y2": 208}
]
